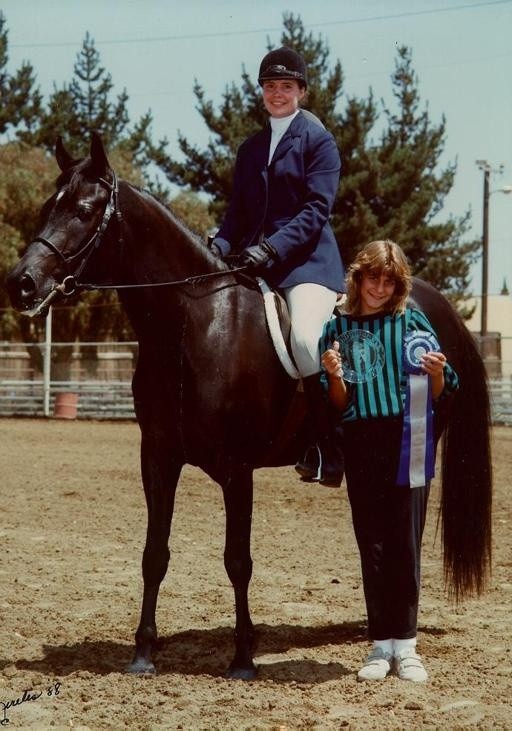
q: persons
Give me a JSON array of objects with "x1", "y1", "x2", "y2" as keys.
[
  {"x1": 318, "y1": 240, "x2": 460, "y2": 684},
  {"x1": 210, "y1": 47, "x2": 344, "y2": 479}
]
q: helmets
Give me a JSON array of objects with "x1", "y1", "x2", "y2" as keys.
[{"x1": 257, "y1": 48, "x2": 308, "y2": 89}]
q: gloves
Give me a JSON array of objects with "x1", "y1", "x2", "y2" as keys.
[
  {"x1": 237, "y1": 240, "x2": 272, "y2": 269},
  {"x1": 210, "y1": 245, "x2": 222, "y2": 260}
]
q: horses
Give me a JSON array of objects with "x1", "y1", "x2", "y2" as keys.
[{"x1": 6, "y1": 131, "x2": 493, "y2": 681}]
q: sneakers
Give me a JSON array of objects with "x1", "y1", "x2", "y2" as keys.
[
  {"x1": 359, "y1": 646, "x2": 394, "y2": 680},
  {"x1": 395, "y1": 649, "x2": 428, "y2": 681}
]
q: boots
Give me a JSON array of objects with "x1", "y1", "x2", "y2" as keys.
[{"x1": 301, "y1": 371, "x2": 343, "y2": 489}]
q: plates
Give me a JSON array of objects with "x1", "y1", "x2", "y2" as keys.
[{"x1": 332, "y1": 329, "x2": 386, "y2": 384}]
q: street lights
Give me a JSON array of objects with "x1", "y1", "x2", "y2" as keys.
[{"x1": 479, "y1": 156, "x2": 511, "y2": 359}]
